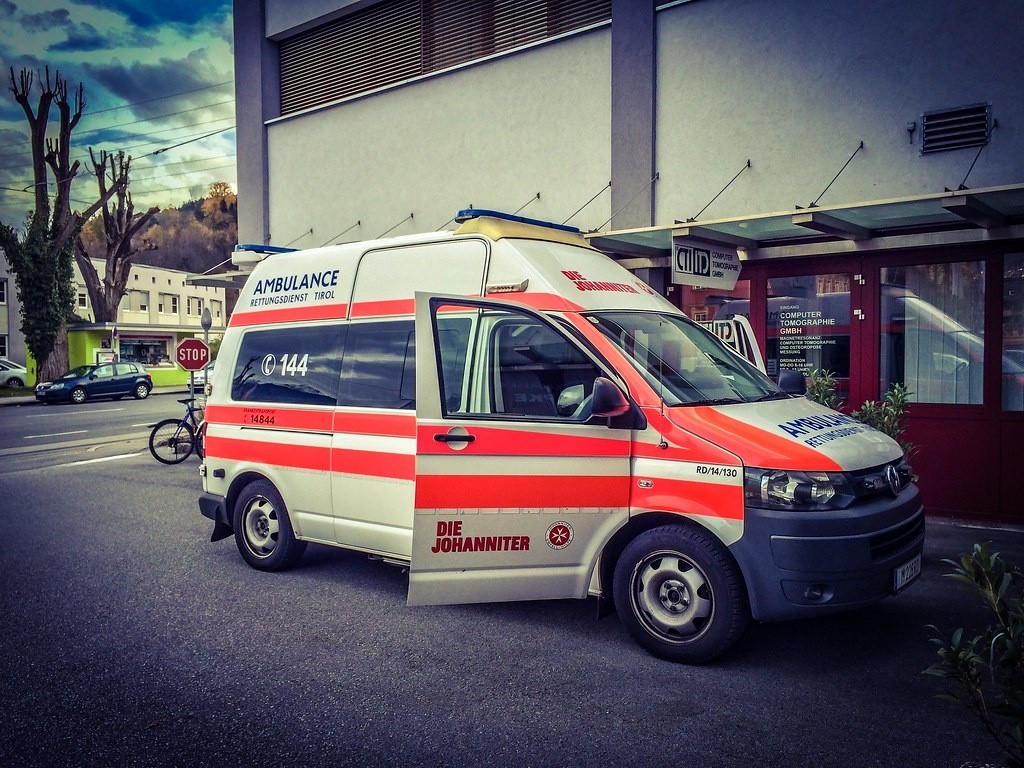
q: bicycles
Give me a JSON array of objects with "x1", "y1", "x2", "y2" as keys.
[{"x1": 149, "y1": 398, "x2": 205, "y2": 465}]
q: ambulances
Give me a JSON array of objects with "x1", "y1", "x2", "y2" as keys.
[
  {"x1": 706, "y1": 280, "x2": 1024, "y2": 410},
  {"x1": 195, "y1": 205, "x2": 927, "y2": 666}
]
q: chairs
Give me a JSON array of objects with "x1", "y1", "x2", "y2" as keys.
[{"x1": 500, "y1": 341, "x2": 559, "y2": 417}]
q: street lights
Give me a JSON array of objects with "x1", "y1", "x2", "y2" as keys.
[{"x1": 200, "y1": 306, "x2": 212, "y2": 399}]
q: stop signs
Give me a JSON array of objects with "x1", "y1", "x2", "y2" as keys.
[{"x1": 175, "y1": 337, "x2": 211, "y2": 371}]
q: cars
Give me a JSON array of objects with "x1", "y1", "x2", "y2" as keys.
[
  {"x1": 34, "y1": 360, "x2": 154, "y2": 404},
  {"x1": 0, "y1": 358, "x2": 28, "y2": 389},
  {"x1": 187, "y1": 359, "x2": 216, "y2": 394}
]
